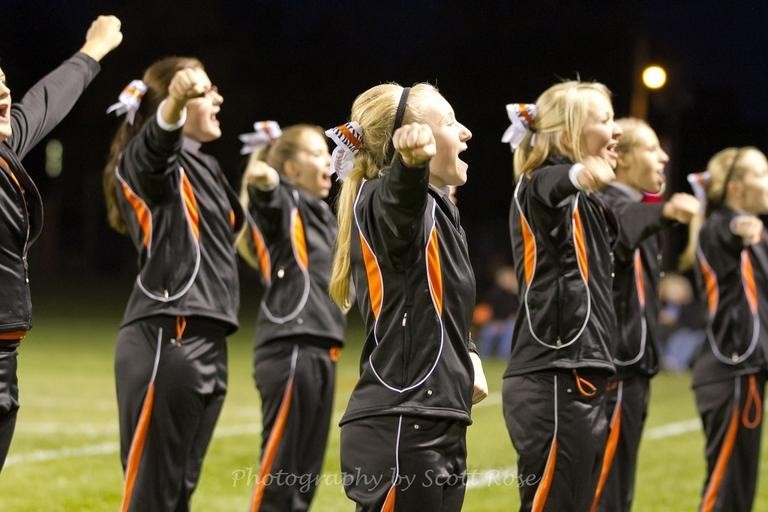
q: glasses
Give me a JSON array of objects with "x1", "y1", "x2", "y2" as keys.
[{"x1": 188, "y1": 84, "x2": 218, "y2": 99}]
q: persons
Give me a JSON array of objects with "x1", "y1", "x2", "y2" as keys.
[
  {"x1": 103, "y1": 52, "x2": 250, "y2": 510},
  {"x1": 328, "y1": 77, "x2": 490, "y2": 512},
  {"x1": 500, "y1": 79, "x2": 702, "y2": 511},
  {"x1": 233, "y1": 118, "x2": 356, "y2": 511},
  {"x1": 592, "y1": 115, "x2": 668, "y2": 511},
  {"x1": 0, "y1": 14, "x2": 124, "y2": 476},
  {"x1": 679, "y1": 144, "x2": 768, "y2": 510}
]
